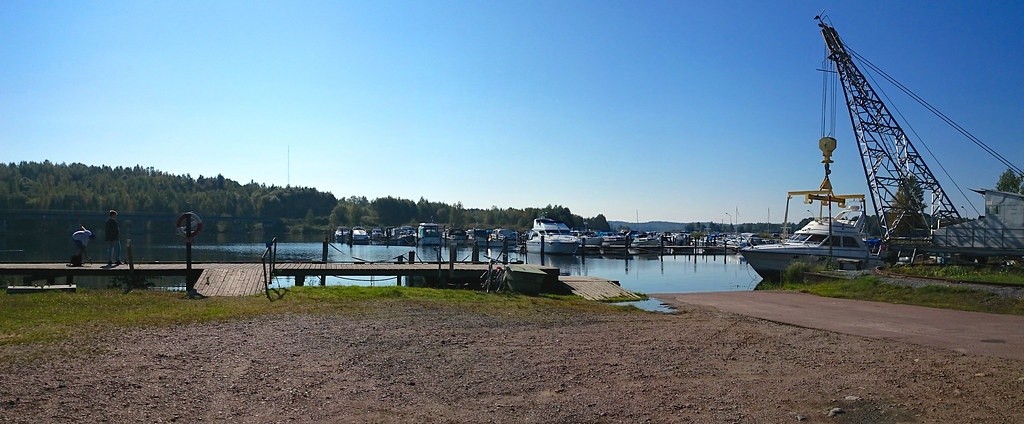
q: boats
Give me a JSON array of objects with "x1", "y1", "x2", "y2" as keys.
[{"x1": 331, "y1": 212, "x2": 1013, "y2": 283}]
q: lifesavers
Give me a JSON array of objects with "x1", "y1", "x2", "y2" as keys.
[{"x1": 177, "y1": 213, "x2": 202, "y2": 239}]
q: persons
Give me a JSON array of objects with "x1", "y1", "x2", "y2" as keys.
[
  {"x1": 71, "y1": 225, "x2": 97, "y2": 266},
  {"x1": 103, "y1": 210, "x2": 124, "y2": 267}
]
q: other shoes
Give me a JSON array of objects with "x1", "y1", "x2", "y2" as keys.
[
  {"x1": 108, "y1": 261, "x2": 114, "y2": 264},
  {"x1": 116, "y1": 261, "x2": 124, "y2": 264}
]
